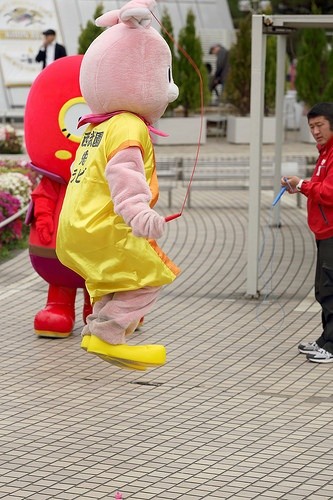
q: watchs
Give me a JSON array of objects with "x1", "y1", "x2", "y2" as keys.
[{"x1": 296, "y1": 179, "x2": 304, "y2": 191}]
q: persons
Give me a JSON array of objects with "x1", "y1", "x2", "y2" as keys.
[
  {"x1": 209, "y1": 43, "x2": 227, "y2": 92},
  {"x1": 281, "y1": 102, "x2": 333, "y2": 364},
  {"x1": 35, "y1": 30, "x2": 67, "y2": 69}
]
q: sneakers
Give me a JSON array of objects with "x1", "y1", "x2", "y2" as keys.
[
  {"x1": 306, "y1": 348, "x2": 333, "y2": 363},
  {"x1": 298, "y1": 341, "x2": 323, "y2": 353}
]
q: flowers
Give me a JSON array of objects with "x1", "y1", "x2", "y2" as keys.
[
  {"x1": 0, "y1": 125, "x2": 24, "y2": 154},
  {"x1": 0, "y1": 159, "x2": 43, "y2": 261}
]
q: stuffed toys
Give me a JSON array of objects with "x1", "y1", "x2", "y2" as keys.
[
  {"x1": 24, "y1": 55, "x2": 144, "y2": 337},
  {"x1": 55, "y1": 0, "x2": 180, "y2": 371}
]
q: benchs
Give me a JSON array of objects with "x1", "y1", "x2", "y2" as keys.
[{"x1": 156, "y1": 155, "x2": 307, "y2": 209}]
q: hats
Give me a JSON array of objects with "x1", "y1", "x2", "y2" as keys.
[{"x1": 43, "y1": 29, "x2": 55, "y2": 35}]
